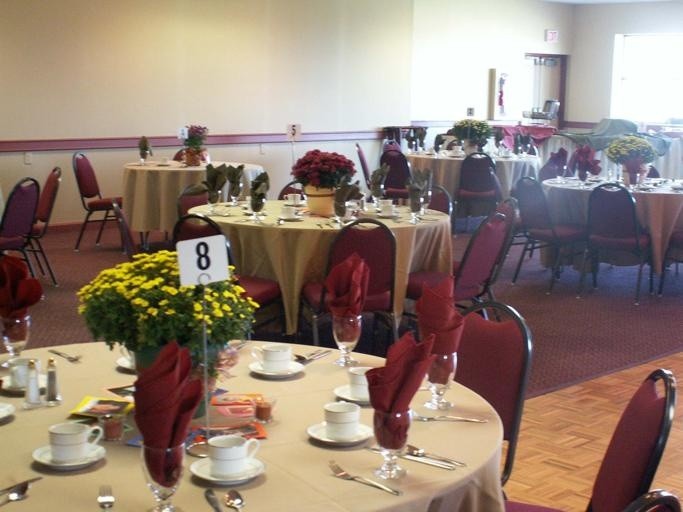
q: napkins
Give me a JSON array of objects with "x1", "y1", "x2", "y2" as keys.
[
  {"x1": 0, "y1": 253, "x2": 45, "y2": 318},
  {"x1": 365, "y1": 331, "x2": 436, "y2": 414},
  {"x1": 132, "y1": 342, "x2": 204, "y2": 449}
]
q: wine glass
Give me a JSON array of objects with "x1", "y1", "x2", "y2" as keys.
[
  {"x1": 553, "y1": 166, "x2": 639, "y2": 188},
  {"x1": 371, "y1": 410, "x2": 410, "y2": 481},
  {"x1": 200, "y1": 183, "x2": 436, "y2": 225},
  {"x1": 138, "y1": 444, "x2": 186, "y2": 512},
  {"x1": 330, "y1": 314, "x2": 361, "y2": 367},
  {"x1": 426, "y1": 352, "x2": 457, "y2": 413},
  {"x1": 2, "y1": 316, "x2": 31, "y2": 370}
]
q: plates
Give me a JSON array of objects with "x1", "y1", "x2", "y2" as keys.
[
  {"x1": 308, "y1": 423, "x2": 373, "y2": 446},
  {"x1": 0, "y1": 375, "x2": 48, "y2": 395},
  {"x1": 31, "y1": 444, "x2": 107, "y2": 472},
  {"x1": 245, "y1": 362, "x2": 306, "y2": 379},
  {"x1": 113, "y1": 355, "x2": 135, "y2": 373},
  {"x1": 0, "y1": 401, "x2": 17, "y2": 421},
  {"x1": 192, "y1": 457, "x2": 263, "y2": 483},
  {"x1": 332, "y1": 385, "x2": 374, "y2": 406}
]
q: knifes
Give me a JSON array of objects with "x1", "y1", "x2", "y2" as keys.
[
  {"x1": 416, "y1": 412, "x2": 487, "y2": 423},
  {"x1": 202, "y1": 488, "x2": 221, "y2": 510},
  {"x1": 363, "y1": 448, "x2": 455, "y2": 471},
  {"x1": 1, "y1": 476, "x2": 43, "y2": 495}
]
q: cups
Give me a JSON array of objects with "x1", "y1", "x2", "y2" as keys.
[
  {"x1": 97, "y1": 413, "x2": 121, "y2": 441},
  {"x1": 6, "y1": 358, "x2": 39, "y2": 388},
  {"x1": 208, "y1": 434, "x2": 258, "y2": 476},
  {"x1": 252, "y1": 395, "x2": 276, "y2": 422},
  {"x1": 423, "y1": 145, "x2": 532, "y2": 158},
  {"x1": 322, "y1": 400, "x2": 360, "y2": 438},
  {"x1": 47, "y1": 422, "x2": 101, "y2": 461},
  {"x1": 250, "y1": 345, "x2": 295, "y2": 374},
  {"x1": 348, "y1": 365, "x2": 379, "y2": 397}
]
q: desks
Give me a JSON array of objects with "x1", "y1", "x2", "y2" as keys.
[{"x1": 0, "y1": 338, "x2": 502, "y2": 512}]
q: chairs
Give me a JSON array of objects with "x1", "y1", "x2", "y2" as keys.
[
  {"x1": 503, "y1": 367, "x2": 677, "y2": 512},
  {"x1": 528, "y1": 98, "x2": 559, "y2": 127},
  {"x1": 623, "y1": 489, "x2": 682, "y2": 512},
  {"x1": 453, "y1": 299, "x2": 533, "y2": 485},
  {"x1": 0, "y1": 166, "x2": 62, "y2": 286},
  {"x1": 72, "y1": 127, "x2": 683, "y2": 356}
]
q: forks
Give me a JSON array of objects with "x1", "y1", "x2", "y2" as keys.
[
  {"x1": 95, "y1": 483, "x2": 115, "y2": 510},
  {"x1": 326, "y1": 457, "x2": 399, "y2": 496}
]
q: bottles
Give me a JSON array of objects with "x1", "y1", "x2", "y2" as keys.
[
  {"x1": 24, "y1": 360, "x2": 41, "y2": 409},
  {"x1": 43, "y1": 359, "x2": 62, "y2": 406}
]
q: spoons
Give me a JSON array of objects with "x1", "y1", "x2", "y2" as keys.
[
  {"x1": 291, "y1": 348, "x2": 330, "y2": 362},
  {"x1": 47, "y1": 348, "x2": 82, "y2": 362},
  {"x1": 223, "y1": 488, "x2": 244, "y2": 512},
  {"x1": 0, "y1": 482, "x2": 28, "y2": 506},
  {"x1": 406, "y1": 443, "x2": 470, "y2": 466}
]
